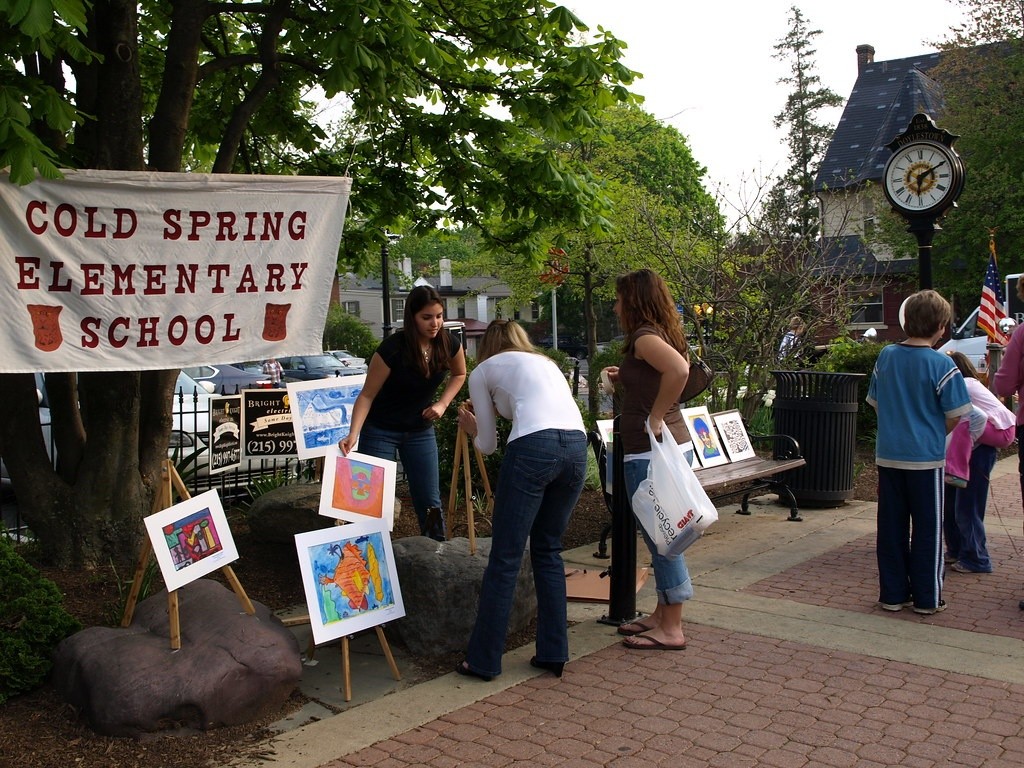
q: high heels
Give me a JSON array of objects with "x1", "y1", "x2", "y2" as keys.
[
  {"x1": 530, "y1": 656, "x2": 565, "y2": 677},
  {"x1": 456, "y1": 660, "x2": 492, "y2": 681}
]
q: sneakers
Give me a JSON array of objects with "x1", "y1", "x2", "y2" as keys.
[
  {"x1": 951, "y1": 561, "x2": 973, "y2": 572},
  {"x1": 944, "y1": 552, "x2": 958, "y2": 563},
  {"x1": 914, "y1": 599, "x2": 947, "y2": 614},
  {"x1": 944, "y1": 474, "x2": 967, "y2": 488},
  {"x1": 881, "y1": 594, "x2": 914, "y2": 611}
]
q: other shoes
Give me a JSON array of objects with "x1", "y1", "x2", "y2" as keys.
[{"x1": 1019, "y1": 600, "x2": 1024, "y2": 609}]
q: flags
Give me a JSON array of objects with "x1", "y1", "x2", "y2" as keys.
[{"x1": 976, "y1": 239, "x2": 1012, "y2": 347}]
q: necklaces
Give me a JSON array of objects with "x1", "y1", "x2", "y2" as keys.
[{"x1": 421, "y1": 342, "x2": 431, "y2": 363}]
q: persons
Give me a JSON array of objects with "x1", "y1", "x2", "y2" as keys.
[
  {"x1": 865, "y1": 290, "x2": 973, "y2": 614},
  {"x1": 456, "y1": 320, "x2": 588, "y2": 682},
  {"x1": 777, "y1": 317, "x2": 805, "y2": 364},
  {"x1": 863, "y1": 327, "x2": 878, "y2": 345},
  {"x1": 263, "y1": 359, "x2": 285, "y2": 389},
  {"x1": 601, "y1": 269, "x2": 694, "y2": 651},
  {"x1": 339, "y1": 286, "x2": 466, "y2": 543},
  {"x1": 993, "y1": 273, "x2": 1024, "y2": 611},
  {"x1": 943, "y1": 350, "x2": 1016, "y2": 574}
]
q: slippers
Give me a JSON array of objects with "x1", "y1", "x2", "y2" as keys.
[{"x1": 617, "y1": 621, "x2": 687, "y2": 650}]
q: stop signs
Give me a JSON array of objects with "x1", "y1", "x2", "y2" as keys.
[{"x1": 537, "y1": 247, "x2": 570, "y2": 287}]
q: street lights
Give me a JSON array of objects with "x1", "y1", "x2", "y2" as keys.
[{"x1": 358, "y1": 219, "x2": 393, "y2": 338}]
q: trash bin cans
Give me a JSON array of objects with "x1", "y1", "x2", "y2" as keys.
[{"x1": 770, "y1": 367, "x2": 867, "y2": 509}]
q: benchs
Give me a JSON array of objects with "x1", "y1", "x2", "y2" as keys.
[{"x1": 588, "y1": 412, "x2": 806, "y2": 567}]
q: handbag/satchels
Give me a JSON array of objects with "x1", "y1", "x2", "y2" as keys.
[
  {"x1": 679, "y1": 342, "x2": 714, "y2": 404},
  {"x1": 632, "y1": 414, "x2": 719, "y2": 561}
]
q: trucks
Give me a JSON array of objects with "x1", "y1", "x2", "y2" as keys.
[{"x1": 937, "y1": 273, "x2": 1024, "y2": 373}]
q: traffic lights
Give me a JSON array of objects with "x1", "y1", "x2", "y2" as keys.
[{"x1": 702, "y1": 302, "x2": 714, "y2": 314}]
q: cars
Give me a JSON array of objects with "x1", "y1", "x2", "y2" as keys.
[
  {"x1": 324, "y1": 351, "x2": 368, "y2": 371},
  {"x1": 182, "y1": 365, "x2": 273, "y2": 396},
  {"x1": 536, "y1": 335, "x2": 589, "y2": 360},
  {"x1": 261, "y1": 353, "x2": 365, "y2": 381},
  {"x1": 596, "y1": 335, "x2": 624, "y2": 353},
  {"x1": 686, "y1": 333, "x2": 723, "y2": 357},
  {"x1": 0, "y1": 371, "x2": 242, "y2": 485}
]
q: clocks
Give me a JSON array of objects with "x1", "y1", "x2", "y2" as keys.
[{"x1": 881, "y1": 140, "x2": 962, "y2": 222}]
